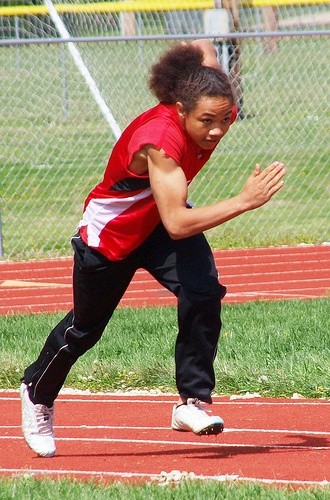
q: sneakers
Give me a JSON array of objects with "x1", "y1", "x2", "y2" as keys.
[
  {"x1": 172, "y1": 400, "x2": 224, "y2": 436},
  {"x1": 19, "y1": 383, "x2": 57, "y2": 457}
]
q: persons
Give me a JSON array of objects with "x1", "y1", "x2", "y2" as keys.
[
  {"x1": 19, "y1": 37, "x2": 286, "y2": 460},
  {"x1": 205, "y1": 1, "x2": 257, "y2": 121}
]
